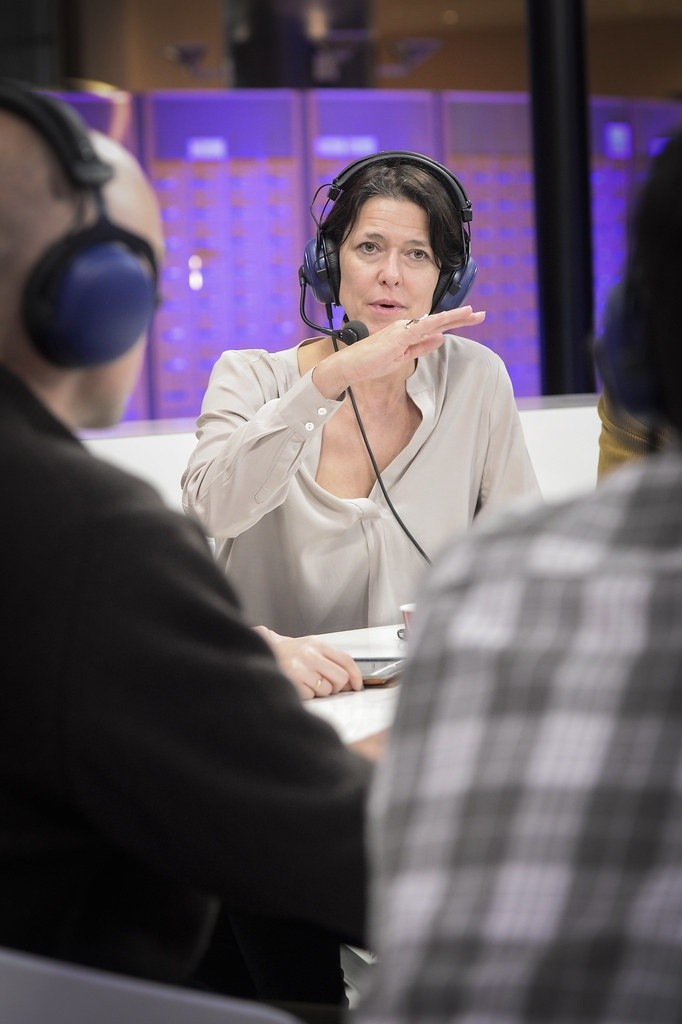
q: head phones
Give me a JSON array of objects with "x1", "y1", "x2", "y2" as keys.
[
  {"x1": 302, "y1": 148, "x2": 478, "y2": 324},
  {"x1": 0, "y1": 76, "x2": 163, "y2": 371}
]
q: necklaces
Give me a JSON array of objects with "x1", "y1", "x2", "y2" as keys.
[{"x1": 178, "y1": 153, "x2": 544, "y2": 606}]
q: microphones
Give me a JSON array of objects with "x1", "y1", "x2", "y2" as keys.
[{"x1": 298, "y1": 283, "x2": 369, "y2": 348}]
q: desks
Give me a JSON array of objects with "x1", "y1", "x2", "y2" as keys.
[{"x1": 237, "y1": 616, "x2": 411, "y2": 753}]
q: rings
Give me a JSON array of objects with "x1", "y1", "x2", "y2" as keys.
[
  {"x1": 403, "y1": 315, "x2": 422, "y2": 328},
  {"x1": 313, "y1": 677, "x2": 327, "y2": 692}
]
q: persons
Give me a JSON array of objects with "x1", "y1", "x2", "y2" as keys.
[
  {"x1": 380, "y1": 130, "x2": 681, "y2": 1023},
  {"x1": 0, "y1": 104, "x2": 370, "y2": 1024},
  {"x1": 226, "y1": 622, "x2": 363, "y2": 700},
  {"x1": 589, "y1": 382, "x2": 675, "y2": 483}
]
